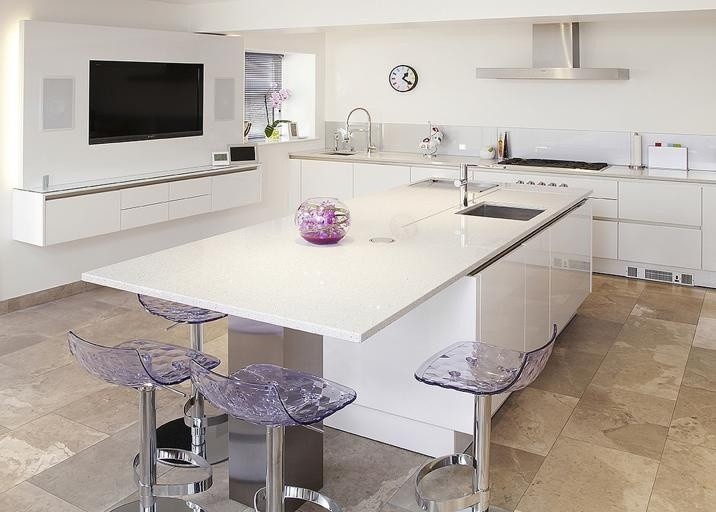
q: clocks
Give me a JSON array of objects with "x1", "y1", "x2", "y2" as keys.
[{"x1": 389, "y1": 65, "x2": 418, "y2": 92}]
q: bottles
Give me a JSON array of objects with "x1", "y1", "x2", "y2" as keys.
[
  {"x1": 497, "y1": 132, "x2": 504, "y2": 160},
  {"x1": 503, "y1": 133, "x2": 510, "y2": 159}
]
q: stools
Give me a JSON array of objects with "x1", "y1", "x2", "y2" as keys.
[
  {"x1": 136, "y1": 293, "x2": 228, "y2": 467},
  {"x1": 413, "y1": 324, "x2": 560, "y2": 511},
  {"x1": 187, "y1": 356, "x2": 356, "y2": 511},
  {"x1": 65, "y1": 330, "x2": 214, "y2": 512}
]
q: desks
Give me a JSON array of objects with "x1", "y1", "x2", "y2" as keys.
[{"x1": 80, "y1": 181, "x2": 596, "y2": 460}]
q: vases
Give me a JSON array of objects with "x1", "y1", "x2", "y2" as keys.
[{"x1": 265, "y1": 125, "x2": 280, "y2": 141}]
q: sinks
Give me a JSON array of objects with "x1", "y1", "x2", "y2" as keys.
[
  {"x1": 453, "y1": 201, "x2": 547, "y2": 223},
  {"x1": 325, "y1": 151, "x2": 353, "y2": 156}
]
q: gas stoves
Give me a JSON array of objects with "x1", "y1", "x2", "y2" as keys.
[{"x1": 491, "y1": 158, "x2": 613, "y2": 192}]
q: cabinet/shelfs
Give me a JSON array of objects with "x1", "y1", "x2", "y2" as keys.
[
  {"x1": 118, "y1": 177, "x2": 212, "y2": 230},
  {"x1": 618, "y1": 177, "x2": 702, "y2": 276},
  {"x1": 353, "y1": 164, "x2": 411, "y2": 196},
  {"x1": 288, "y1": 159, "x2": 354, "y2": 214},
  {"x1": 208, "y1": 166, "x2": 263, "y2": 213},
  {"x1": 13, "y1": 191, "x2": 120, "y2": 247},
  {"x1": 410, "y1": 167, "x2": 474, "y2": 188}
]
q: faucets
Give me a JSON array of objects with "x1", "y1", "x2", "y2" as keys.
[
  {"x1": 346, "y1": 106, "x2": 373, "y2": 153},
  {"x1": 459, "y1": 161, "x2": 477, "y2": 206}
]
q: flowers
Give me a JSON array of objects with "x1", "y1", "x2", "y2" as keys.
[{"x1": 260, "y1": 83, "x2": 293, "y2": 138}]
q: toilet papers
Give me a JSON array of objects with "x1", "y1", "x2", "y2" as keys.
[{"x1": 630, "y1": 131, "x2": 644, "y2": 168}]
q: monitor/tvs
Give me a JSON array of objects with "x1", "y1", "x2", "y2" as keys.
[{"x1": 89, "y1": 59, "x2": 204, "y2": 146}]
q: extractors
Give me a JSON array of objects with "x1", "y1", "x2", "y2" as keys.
[{"x1": 475, "y1": 23, "x2": 630, "y2": 81}]
